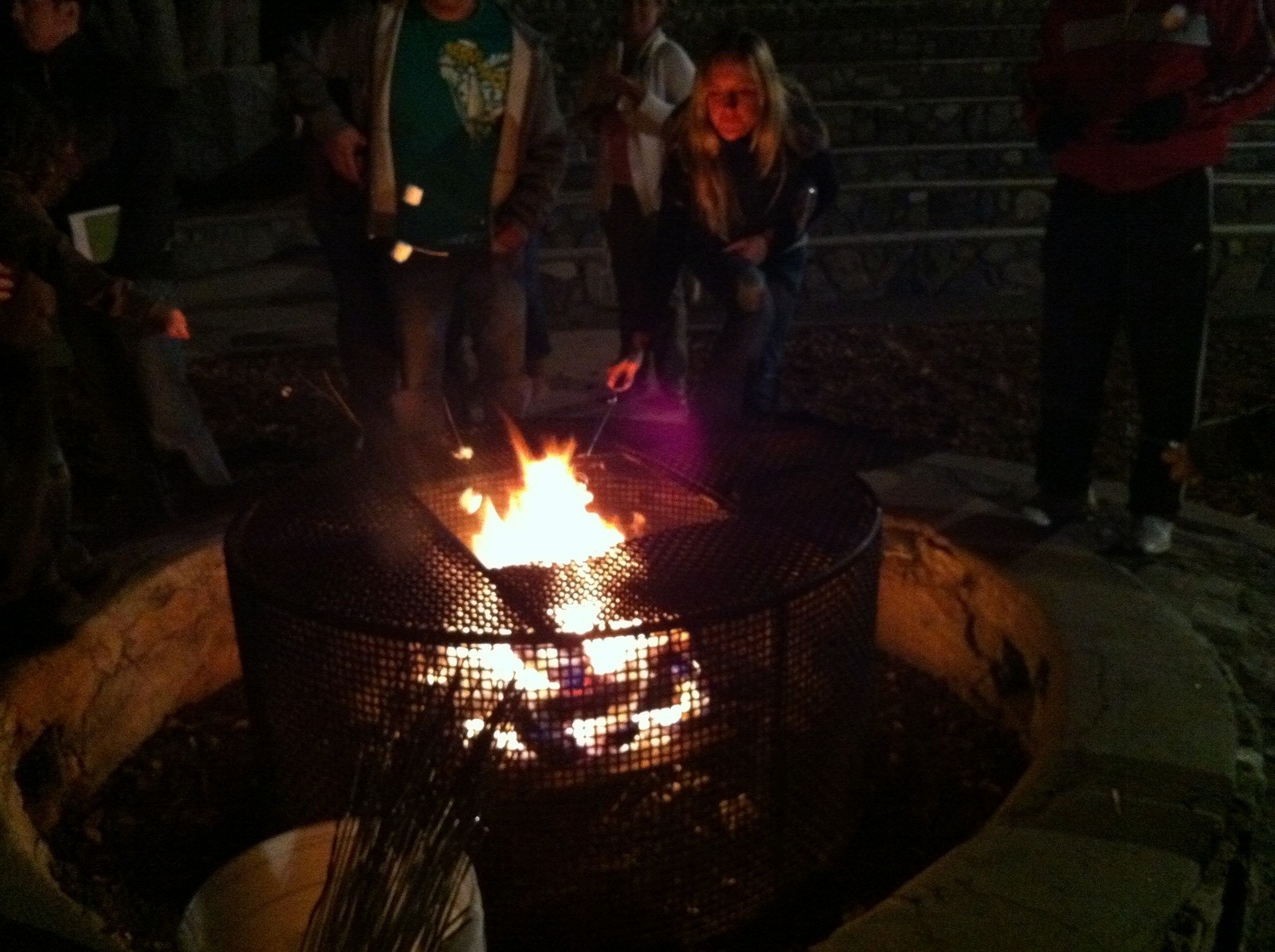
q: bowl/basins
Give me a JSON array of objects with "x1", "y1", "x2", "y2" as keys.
[{"x1": 173, "y1": 816, "x2": 487, "y2": 952}]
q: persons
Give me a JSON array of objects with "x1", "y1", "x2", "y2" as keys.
[
  {"x1": 0, "y1": 0, "x2": 236, "y2": 652},
  {"x1": 657, "y1": 26, "x2": 840, "y2": 423},
  {"x1": 299, "y1": 1, "x2": 572, "y2": 428},
  {"x1": 1011, "y1": 0, "x2": 1275, "y2": 558},
  {"x1": 571, "y1": 0, "x2": 696, "y2": 391}
]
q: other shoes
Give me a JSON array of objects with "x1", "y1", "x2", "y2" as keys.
[
  {"x1": 1019, "y1": 486, "x2": 1095, "y2": 530},
  {"x1": 1135, "y1": 515, "x2": 1172, "y2": 559}
]
